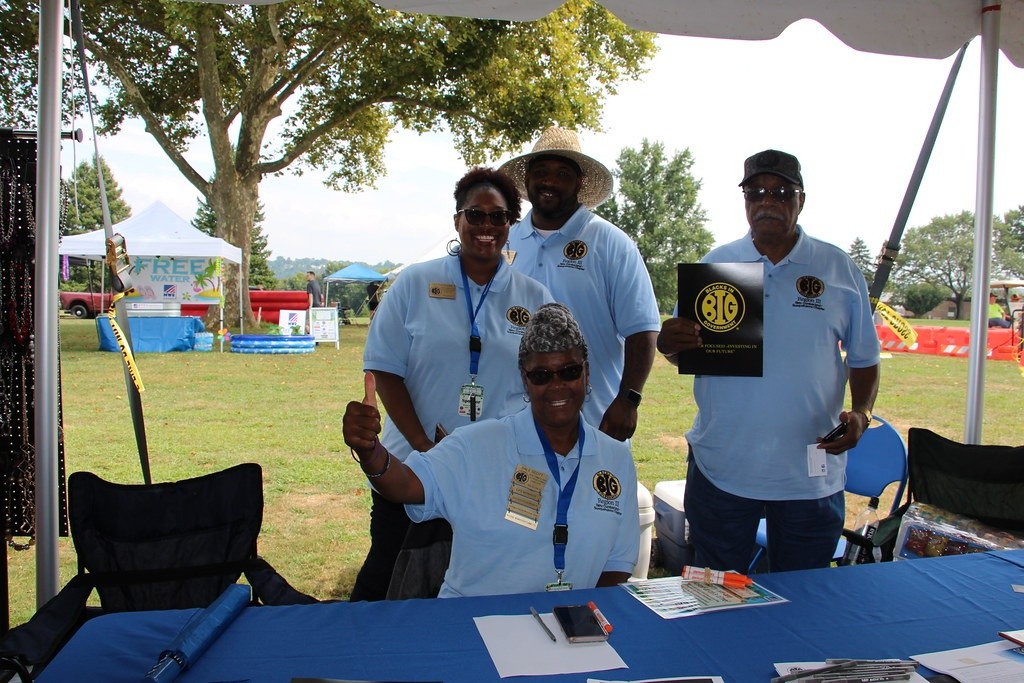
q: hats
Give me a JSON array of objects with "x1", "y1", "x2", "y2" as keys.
[
  {"x1": 497, "y1": 126, "x2": 613, "y2": 210},
  {"x1": 739, "y1": 149, "x2": 804, "y2": 188}
]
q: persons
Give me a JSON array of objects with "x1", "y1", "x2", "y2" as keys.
[
  {"x1": 988, "y1": 292, "x2": 1015, "y2": 328},
  {"x1": 366, "y1": 282, "x2": 380, "y2": 324},
  {"x1": 658, "y1": 150, "x2": 881, "y2": 573},
  {"x1": 1008, "y1": 294, "x2": 1022, "y2": 318},
  {"x1": 342, "y1": 304, "x2": 640, "y2": 599},
  {"x1": 306, "y1": 271, "x2": 321, "y2": 308},
  {"x1": 348, "y1": 169, "x2": 556, "y2": 602},
  {"x1": 499, "y1": 126, "x2": 662, "y2": 447}
]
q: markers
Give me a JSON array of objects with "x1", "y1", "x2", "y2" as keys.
[
  {"x1": 683, "y1": 565, "x2": 747, "y2": 581},
  {"x1": 681, "y1": 572, "x2": 745, "y2": 588},
  {"x1": 744, "y1": 578, "x2": 752, "y2": 585},
  {"x1": 588, "y1": 601, "x2": 613, "y2": 632}
]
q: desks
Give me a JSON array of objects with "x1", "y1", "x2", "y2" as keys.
[
  {"x1": 34, "y1": 549, "x2": 1023, "y2": 683},
  {"x1": 96, "y1": 314, "x2": 205, "y2": 352}
]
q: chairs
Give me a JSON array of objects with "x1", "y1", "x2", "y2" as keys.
[
  {"x1": 386, "y1": 517, "x2": 453, "y2": 601},
  {"x1": 749, "y1": 415, "x2": 910, "y2": 573},
  {"x1": 838, "y1": 427, "x2": 1024, "y2": 568},
  {"x1": 0, "y1": 463, "x2": 321, "y2": 683}
]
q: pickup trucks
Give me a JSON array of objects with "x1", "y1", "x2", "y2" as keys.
[{"x1": 60, "y1": 292, "x2": 113, "y2": 319}]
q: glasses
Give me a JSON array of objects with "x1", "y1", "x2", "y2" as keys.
[
  {"x1": 742, "y1": 183, "x2": 801, "y2": 203},
  {"x1": 456, "y1": 207, "x2": 512, "y2": 228},
  {"x1": 521, "y1": 359, "x2": 587, "y2": 386}
]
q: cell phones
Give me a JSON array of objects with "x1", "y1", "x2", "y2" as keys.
[
  {"x1": 552, "y1": 605, "x2": 609, "y2": 642},
  {"x1": 823, "y1": 422, "x2": 848, "y2": 443}
]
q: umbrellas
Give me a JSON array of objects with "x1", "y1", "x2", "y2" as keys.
[{"x1": 990, "y1": 278, "x2": 1024, "y2": 293}]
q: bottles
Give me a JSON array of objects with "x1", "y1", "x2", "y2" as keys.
[{"x1": 838, "y1": 496, "x2": 879, "y2": 567}]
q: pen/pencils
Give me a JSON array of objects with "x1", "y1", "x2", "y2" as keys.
[
  {"x1": 821, "y1": 674, "x2": 910, "y2": 683},
  {"x1": 797, "y1": 673, "x2": 888, "y2": 683},
  {"x1": 770, "y1": 661, "x2": 857, "y2": 683},
  {"x1": 530, "y1": 607, "x2": 556, "y2": 642},
  {"x1": 825, "y1": 659, "x2": 920, "y2": 665},
  {"x1": 822, "y1": 666, "x2": 915, "y2": 673},
  {"x1": 813, "y1": 669, "x2": 906, "y2": 677},
  {"x1": 855, "y1": 663, "x2": 917, "y2": 667}
]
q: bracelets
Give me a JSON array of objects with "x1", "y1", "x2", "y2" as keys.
[
  {"x1": 350, "y1": 436, "x2": 379, "y2": 466},
  {"x1": 852, "y1": 407, "x2": 873, "y2": 432},
  {"x1": 360, "y1": 447, "x2": 391, "y2": 478}
]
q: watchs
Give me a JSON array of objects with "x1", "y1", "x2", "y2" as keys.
[{"x1": 617, "y1": 387, "x2": 642, "y2": 406}]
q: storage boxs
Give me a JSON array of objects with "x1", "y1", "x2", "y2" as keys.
[{"x1": 652, "y1": 478, "x2": 690, "y2": 571}]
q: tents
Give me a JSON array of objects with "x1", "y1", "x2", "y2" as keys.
[
  {"x1": 58, "y1": 200, "x2": 242, "y2": 353},
  {"x1": 323, "y1": 263, "x2": 389, "y2": 307},
  {"x1": 34, "y1": 0, "x2": 1024, "y2": 609}
]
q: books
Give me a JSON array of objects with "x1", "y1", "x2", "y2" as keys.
[{"x1": 997, "y1": 629, "x2": 1024, "y2": 648}]
